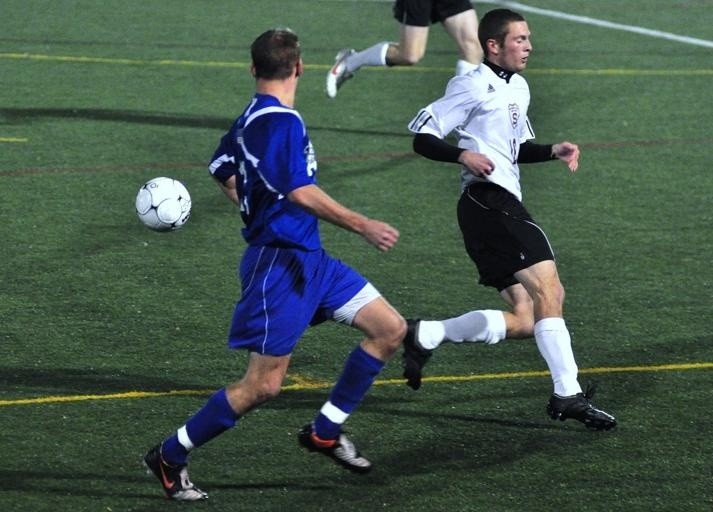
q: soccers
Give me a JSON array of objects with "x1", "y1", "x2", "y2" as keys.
[{"x1": 134, "y1": 176, "x2": 191, "y2": 232}]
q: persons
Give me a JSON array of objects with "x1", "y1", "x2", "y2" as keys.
[
  {"x1": 140, "y1": 26, "x2": 408, "y2": 503},
  {"x1": 323, "y1": 0, "x2": 485, "y2": 101},
  {"x1": 400, "y1": 7, "x2": 619, "y2": 433}
]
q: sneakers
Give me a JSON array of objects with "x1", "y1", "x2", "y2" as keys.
[
  {"x1": 298, "y1": 422, "x2": 373, "y2": 474},
  {"x1": 403, "y1": 318, "x2": 433, "y2": 391},
  {"x1": 546, "y1": 394, "x2": 618, "y2": 431},
  {"x1": 142, "y1": 440, "x2": 209, "y2": 500},
  {"x1": 325, "y1": 49, "x2": 356, "y2": 99}
]
